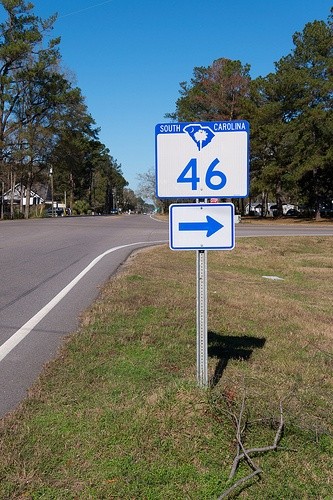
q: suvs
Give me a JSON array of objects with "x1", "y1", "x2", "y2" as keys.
[
  {"x1": 47, "y1": 207, "x2": 64, "y2": 217},
  {"x1": 110, "y1": 209, "x2": 118, "y2": 215}
]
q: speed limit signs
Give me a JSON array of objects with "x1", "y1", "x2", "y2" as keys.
[{"x1": 156, "y1": 121, "x2": 249, "y2": 199}]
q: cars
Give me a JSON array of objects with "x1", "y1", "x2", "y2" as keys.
[{"x1": 246, "y1": 202, "x2": 330, "y2": 218}]
